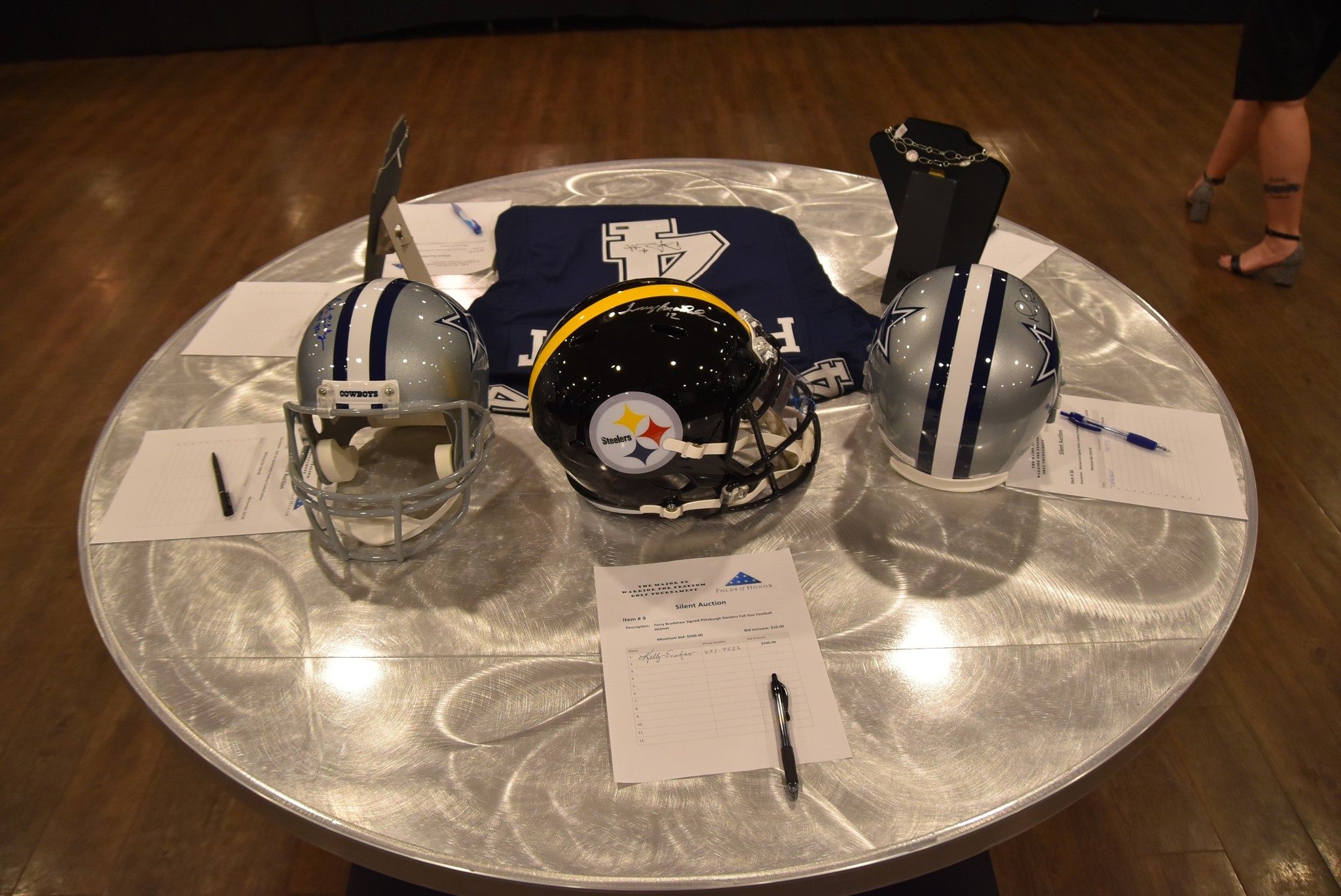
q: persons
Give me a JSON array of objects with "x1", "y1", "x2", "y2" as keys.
[{"x1": 1187, "y1": 0, "x2": 1341, "y2": 289}]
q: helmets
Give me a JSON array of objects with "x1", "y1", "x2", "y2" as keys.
[
  {"x1": 528, "y1": 277, "x2": 821, "y2": 519},
  {"x1": 283, "y1": 277, "x2": 492, "y2": 562},
  {"x1": 863, "y1": 264, "x2": 1066, "y2": 493}
]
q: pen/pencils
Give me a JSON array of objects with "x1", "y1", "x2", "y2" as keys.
[
  {"x1": 771, "y1": 673, "x2": 799, "y2": 801},
  {"x1": 211, "y1": 452, "x2": 234, "y2": 517},
  {"x1": 1061, "y1": 411, "x2": 1172, "y2": 453},
  {"x1": 452, "y1": 202, "x2": 482, "y2": 235}
]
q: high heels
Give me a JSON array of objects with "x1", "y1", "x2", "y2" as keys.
[
  {"x1": 1218, "y1": 225, "x2": 1303, "y2": 287},
  {"x1": 1186, "y1": 172, "x2": 1226, "y2": 222}
]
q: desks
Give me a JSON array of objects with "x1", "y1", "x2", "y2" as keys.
[{"x1": 76, "y1": 154, "x2": 1260, "y2": 896}]
